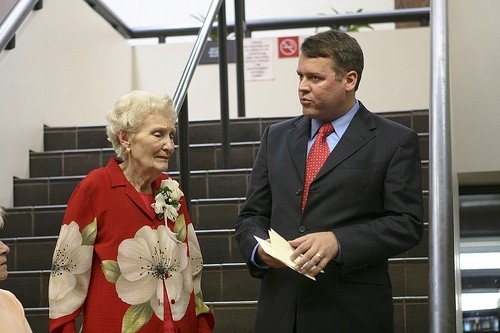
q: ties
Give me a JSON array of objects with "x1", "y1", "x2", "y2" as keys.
[{"x1": 302, "y1": 123, "x2": 334, "y2": 213}]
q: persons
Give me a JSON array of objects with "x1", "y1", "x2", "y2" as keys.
[
  {"x1": 49, "y1": 91, "x2": 215, "y2": 333},
  {"x1": 235, "y1": 29, "x2": 424, "y2": 333}
]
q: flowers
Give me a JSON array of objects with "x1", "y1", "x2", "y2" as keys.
[{"x1": 151, "y1": 176, "x2": 185, "y2": 245}]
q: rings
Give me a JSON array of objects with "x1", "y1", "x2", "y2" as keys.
[{"x1": 315, "y1": 253, "x2": 323, "y2": 259}]
